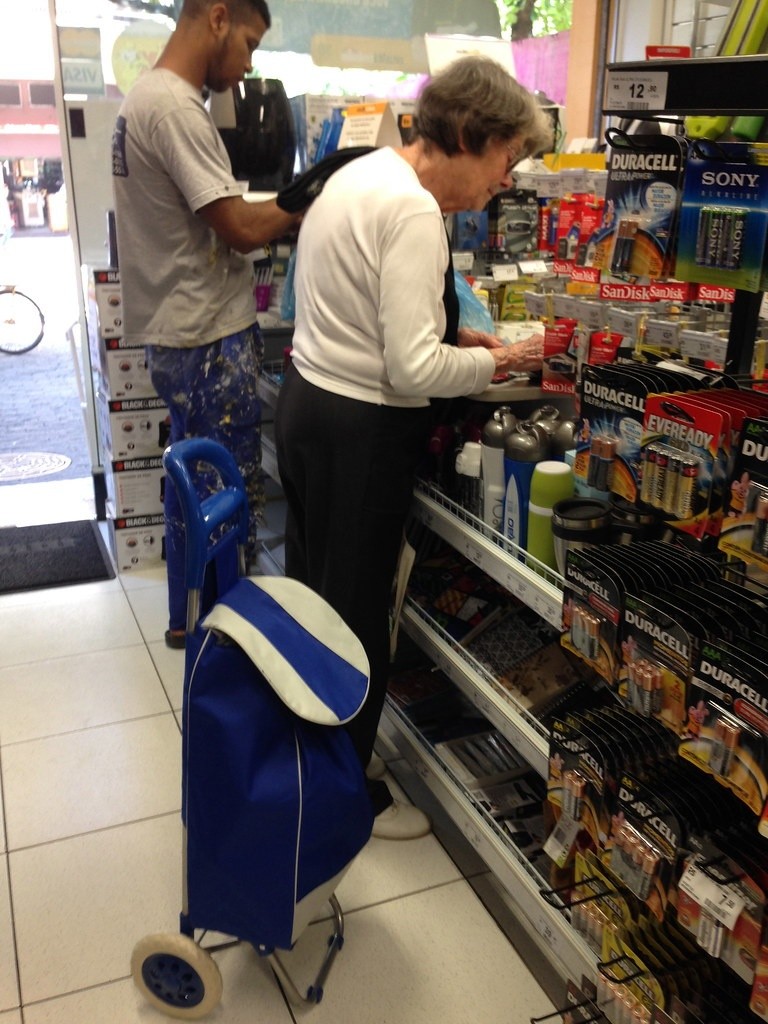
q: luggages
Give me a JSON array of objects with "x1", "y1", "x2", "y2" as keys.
[{"x1": 129, "y1": 434, "x2": 375, "y2": 1019}]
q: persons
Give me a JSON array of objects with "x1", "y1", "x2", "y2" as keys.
[
  {"x1": 272, "y1": 56, "x2": 550, "y2": 841},
  {"x1": 110, "y1": 0, "x2": 302, "y2": 649}
]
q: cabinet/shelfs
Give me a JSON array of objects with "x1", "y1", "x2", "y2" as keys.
[{"x1": 250, "y1": 368, "x2": 655, "y2": 1024}]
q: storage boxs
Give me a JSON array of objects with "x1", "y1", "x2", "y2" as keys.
[
  {"x1": 88, "y1": 335, "x2": 158, "y2": 400},
  {"x1": 95, "y1": 392, "x2": 171, "y2": 461},
  {"x1": 289, "y1": 92, "x2": 347, "y2": 173},
  {"x1": 336, "y1": 102, "x2": 403, "y2": 149},
  {"x1": 105, "y1": 500, "x2": 166, "y2": 575},
  {"x1": 80, "y1": 263, "x2": 124, "y2": 339},
  {"x1": 100, "y1": 445, "x2": 167, "y2": 518}
]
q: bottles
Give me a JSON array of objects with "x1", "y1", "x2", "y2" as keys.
[{"x1": 476, "y1": 404, "x2": 655, "y2": 585}]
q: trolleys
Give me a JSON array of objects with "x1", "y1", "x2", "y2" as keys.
[{"x1": 128, "y1": 436, "x2": 372, "y2": 1021}]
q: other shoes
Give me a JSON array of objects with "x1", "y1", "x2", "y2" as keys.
[{"x1": 165, "y1": 629, "x2": 187, "y2": 650}]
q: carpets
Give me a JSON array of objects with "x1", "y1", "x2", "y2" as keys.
[{"x1": 0, "y1": 520, "x2": 116, "y2": 596}]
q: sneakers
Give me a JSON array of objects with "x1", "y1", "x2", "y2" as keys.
[{"x1": 371, "y1": 798, "x2": 431, "y2": 841}]
